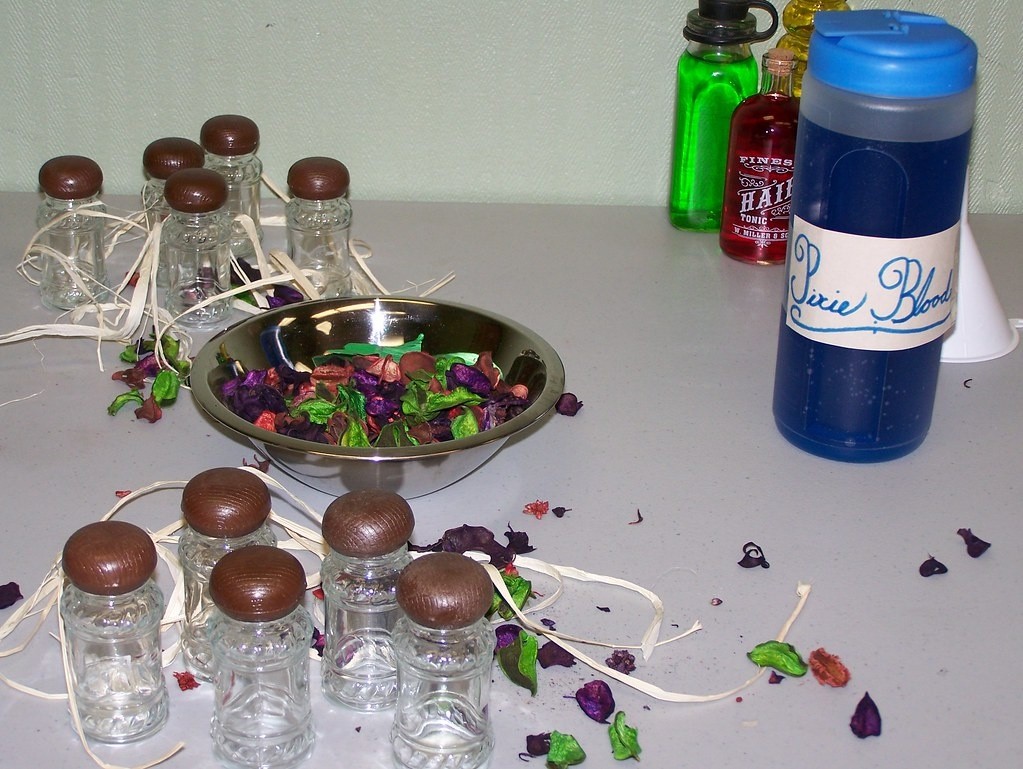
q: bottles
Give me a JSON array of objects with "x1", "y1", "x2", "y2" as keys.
[
  {"x1": 61, "y1": 521, "x2": 167, "y2": 743},
  {"x1": 668, "y1": 1, "x2": 779, "y2": 232},
  {"x1": 287, "y1": 157, "x2": 352, "y2": 300},
  {"x1": 775, "y1": 0, "x2": 851, "y2": 98},
  {"x1": 36, "y1": 155, "x2": 110, "y2": 310},
  {"x1": 200, "y1": 115, "x2": 264, "y2": 258},
  {"x1": 161, "y1": 168, "x2": 232, "y2": 329},
  {"x1": 208, "y1": 545, "x2": 314, "y2": 769},
  {"x1": 719, "y1": 47, "x2": 801, "y2": 267},
  {"x1": 177, "y1": 467, "x2": 276, "y2": 681},
  {"x1": 774, "y1": 10, "x2": 978, "y2": 464},
  {"x1": 144, "y1": 136, "x2": 205, "y2": 288},
  {"x1": 320, "y1": 488, "x2": 413, "y2": 712},
  {"x1": 391, "y1": 552, "x2": 495, "y2": 769}
]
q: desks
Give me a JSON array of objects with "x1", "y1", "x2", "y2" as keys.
[{"x1": 1, "y1": 190, "x2": 1022, "y2": 769}]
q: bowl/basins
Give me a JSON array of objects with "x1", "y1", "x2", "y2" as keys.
[{"x1": 189, "y1": 295, "x2": 566, "y2": 500}]
